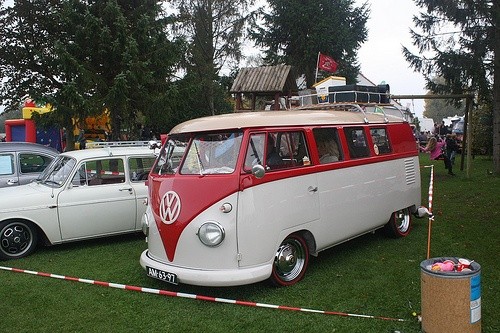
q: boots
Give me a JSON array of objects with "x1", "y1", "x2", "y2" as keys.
[{"x1": 449, "y1": 165, "x2": 456, "y2": 176}]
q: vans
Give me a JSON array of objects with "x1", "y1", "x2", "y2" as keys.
[{"x1": 138, "y1": 109, "x2": 422, "y2": 288}]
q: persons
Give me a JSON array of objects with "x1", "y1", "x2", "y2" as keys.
[
  {"x1": 316, "y1": 140, "x2": 339, "y2": 164},
  {"x1": 417, "y1": 121, "x2": 462, "y2": 175},
  {"x1": 78, "y1": 128, "x2": 113, "y2": 150},
  {"x1": 252, "y1": 134, "x2": 285, "y2": 170}
]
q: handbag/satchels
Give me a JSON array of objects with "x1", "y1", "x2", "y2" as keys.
[{"x1": 378, "y1": 84, "x2": 390, "y2": 103}]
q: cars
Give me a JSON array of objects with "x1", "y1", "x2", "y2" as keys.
[{"x1": 0, "y1": 142, "x2": 92, "y2": 188}]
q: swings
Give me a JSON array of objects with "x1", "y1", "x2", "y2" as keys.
[{"x1": 412, "y1": 99, "x2": 444, "y2": 161}]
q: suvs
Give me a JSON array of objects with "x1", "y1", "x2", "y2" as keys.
[{"x1": 0, "y1": 141, "x2": 187, "y2": 262}]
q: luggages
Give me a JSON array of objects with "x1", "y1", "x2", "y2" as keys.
[{"x1": 329, "y1": 85, "x2": 380, "y2": 102}]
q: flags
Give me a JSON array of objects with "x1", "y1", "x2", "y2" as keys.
[{"x1": 318, "y1": 53, "x2": 339, "y2": 73}]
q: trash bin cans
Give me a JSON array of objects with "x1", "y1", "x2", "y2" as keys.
[{"x1": 418, "y1": 255, "x2": 482, "y2": 333}]
